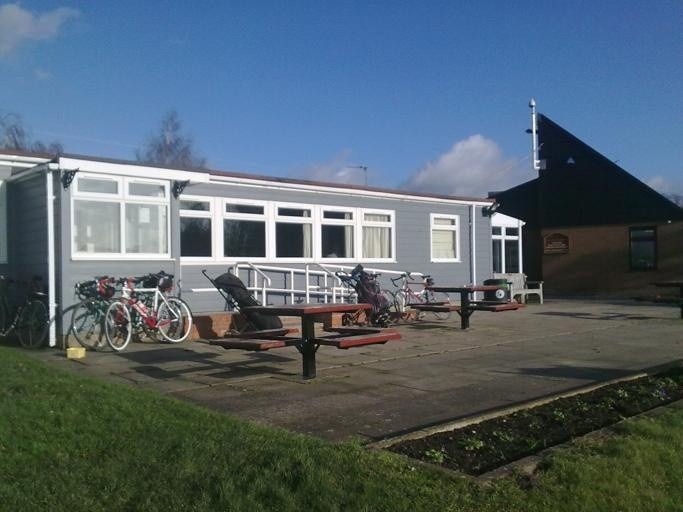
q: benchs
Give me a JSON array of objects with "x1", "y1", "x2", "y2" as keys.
[
  {"x1": 407, "y1": 301, "x2": 526, "y2": 313},
  {"x1": 209, "y1": 326, "x2": 401, "y2": 352},
  {"x1": 493, "y1": 272, "x2": 544, "y2": 304}
]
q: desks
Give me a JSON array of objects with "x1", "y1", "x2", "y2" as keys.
[
  {"x1": 425, "y1": 285, "x2": 503, "y2": 330},
  {"x1": 243, "y1": 302, "x2": 372, "y2": 380}
]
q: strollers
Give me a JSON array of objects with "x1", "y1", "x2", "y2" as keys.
[
  {"x1": 334, "y1": 265, "x2": 394, "y2": 329},
  {"x1": 201, "y1": 267, "x2": 282, "y2": 335}
]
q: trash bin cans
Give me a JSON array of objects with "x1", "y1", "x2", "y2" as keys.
[{"x1": 484, "y1": 279, "x2": 507, "y2": 304}]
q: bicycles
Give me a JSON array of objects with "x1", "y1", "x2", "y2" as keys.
[
  {"x1": 0, "y1": 272, "x2": 51, "y2": 350},
  {"x1": 389, "y1": 270, "x2": 452, "y2": 322},
  {"x1": 70, "y1": 270, "x2": 194, "y2": 351}
]
